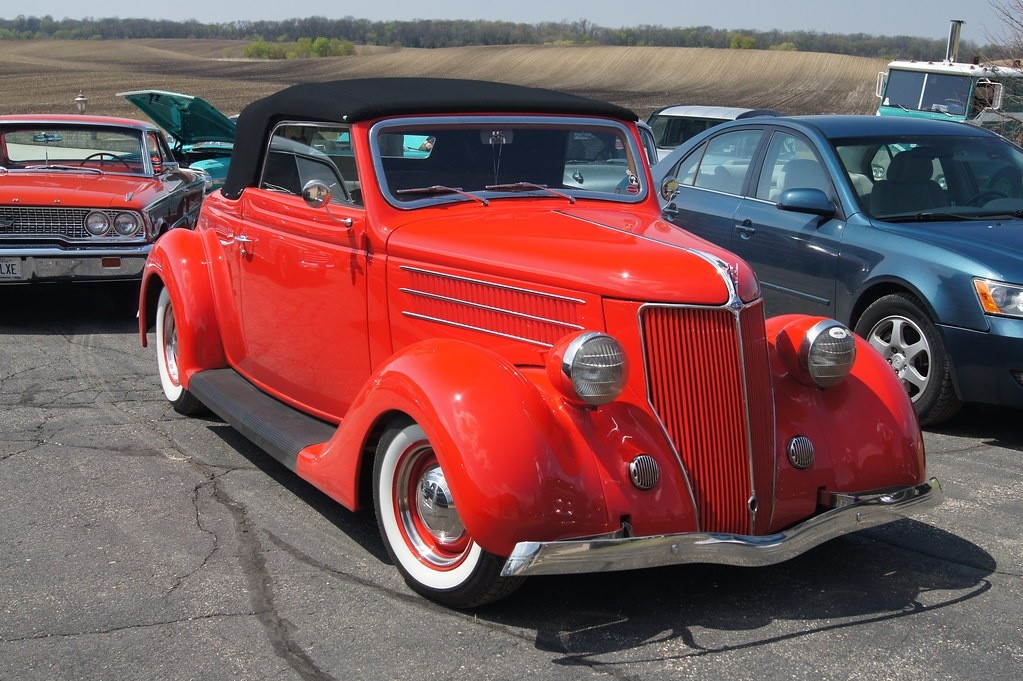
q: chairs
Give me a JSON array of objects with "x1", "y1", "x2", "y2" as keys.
[
  {"x1": 770, "y1": 158, "x2": 834, "y2": 202},
  {"x1": 870, "y1": 151, "x2": 950, "y2": 215}
]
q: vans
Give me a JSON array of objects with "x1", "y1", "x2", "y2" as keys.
[{"x1": 871, "y1": 58, "x2": 1023, "y2": 190}]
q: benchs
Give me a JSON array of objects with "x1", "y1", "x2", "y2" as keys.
[
  {"x1": 294, "y1": 168, "x2": 470, "y2": 203},
  {"x1": 687, "y1": 166, "x2": 873, "y2": 196}
]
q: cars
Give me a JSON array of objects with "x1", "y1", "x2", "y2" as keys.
[
  {"x1": 136, "y1": 77, "x2": 945, "y2": 610},
  {"x1": 107, "y1": 89, "x2": 329, "y2": 198},
  {"x1": 333, "y1": 123, "x2": 436, "y2": 159},
  {"x1": 0, "y1": 112, "x2": 209, "y2": 286},
  {"x1": 614, "y1": 115, "x2": 1023, "y2": 430},
  {"x1": 639, "y1": 103, "x2": 799, "y2": 183}
]
